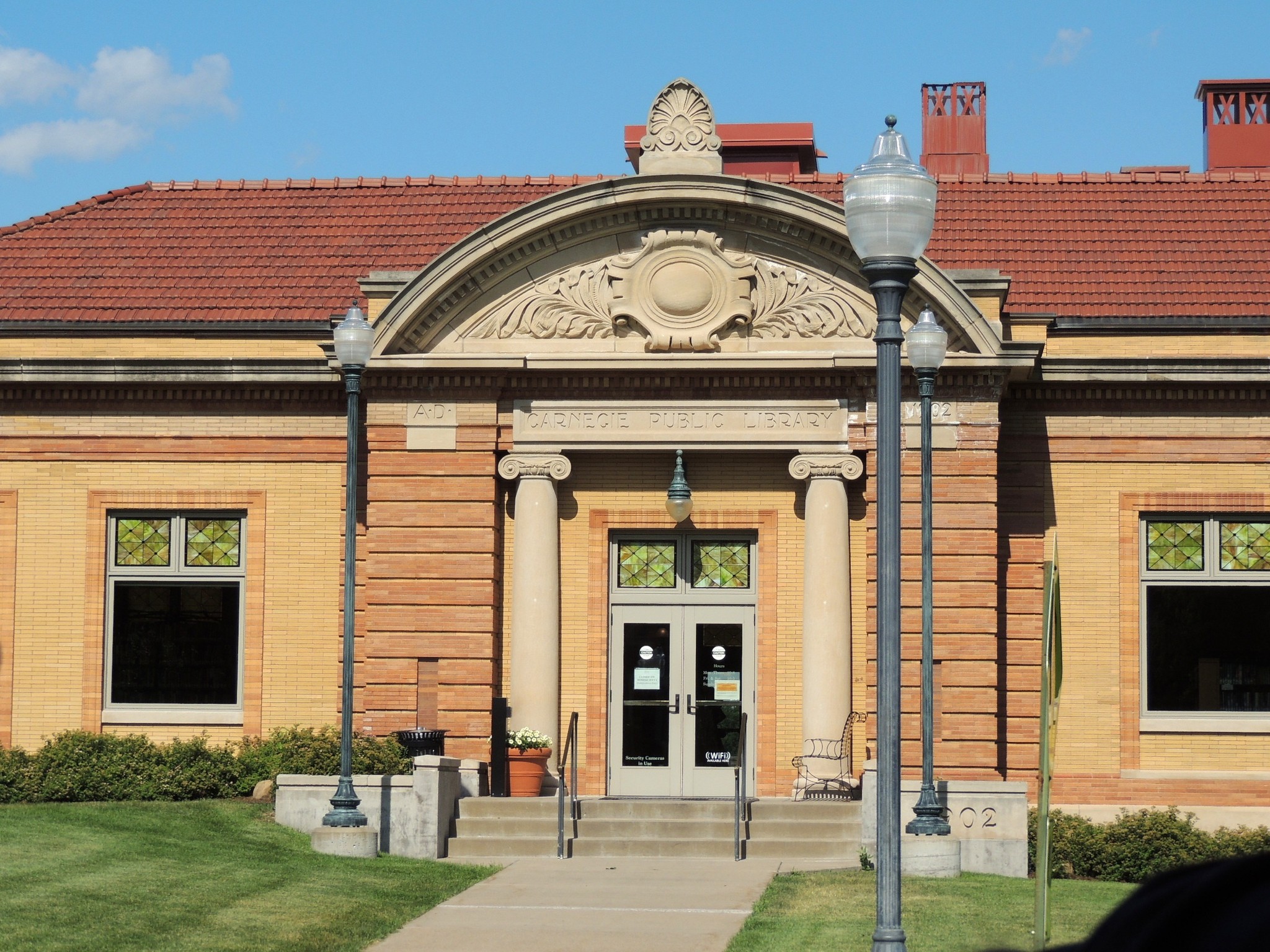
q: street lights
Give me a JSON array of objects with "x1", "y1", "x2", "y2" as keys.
[
  {"x1": 899, "y1": 302, "x2": 961, "y2": 878},
  {"x1": 842, "y1": 116, "x2": 937, "y2": 952},
  {"x1": 311, "y1": 300, "x2": 378, "y2": 859}
]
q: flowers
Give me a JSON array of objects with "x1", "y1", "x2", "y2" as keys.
[{"x1": 504, "y1": 727, "x2": 552, "y2": 751}]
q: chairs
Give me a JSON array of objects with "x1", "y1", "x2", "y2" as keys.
[{"x1": 792, "y1": 711, "x2": 867, "y2": 803}]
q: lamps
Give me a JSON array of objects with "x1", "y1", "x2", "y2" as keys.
[{"x1": 665, "y1": 449, "x2": 693, "y2": 523}]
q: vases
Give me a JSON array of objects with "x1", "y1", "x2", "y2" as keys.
[{"x1": 504, "y1": 747, "x2": 552, "y2": 798}]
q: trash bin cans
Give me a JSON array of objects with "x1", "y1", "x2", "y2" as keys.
[{"x1": 393, "y1": 729, "x2": 451, "y2": 771}]
